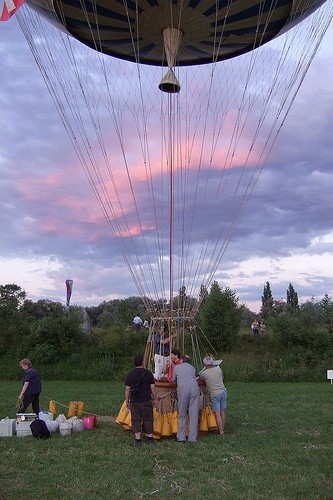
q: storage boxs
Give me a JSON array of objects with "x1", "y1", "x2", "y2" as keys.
[
  {"x1": 0, "y1": 416, "x2": 16, "y2": 437},
  {"x1": 16, "y1": 413, "x2": 37, "y2": 437}
]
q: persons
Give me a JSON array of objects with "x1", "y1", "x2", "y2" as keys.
[
  {"x1": 154, "y1": 327, "x2": 176, "y2": 380},
  {"x1": 133, "y1": 314, "x2": 143, "y2": 331},
  {"x1": 18, "y1": 358, "x2": 40, "y2": 416},
  {"x1": 260, "y1": 321, "x2": 265, "y2": 336},
  {"x1": 200, "y1": 357, "x2": 228, "y2": 434},
  {"x1": 143, "y1": 319, "x2": 149, "y2": 328},
  {"x1": 251, "y1": 318, "x2": 259, "y2": 335},
  {"x1": 125, "y1": 356, "x2": 155, "y2": 442},
  {"x1": 172, "y1": 356, "x2": 200, "y2": 443},
  {"x1": 163, "y1": 348, "x2": 180, "y2": 381}
]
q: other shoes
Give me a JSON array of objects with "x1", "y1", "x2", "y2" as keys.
[
  {"x1": 135, "y1": 439, "x2": 141, "y2": 445},
  {"x1": 148, "y1": 437, "x2": 153, "y2": 443}
]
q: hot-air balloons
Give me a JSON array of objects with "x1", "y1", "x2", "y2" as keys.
[{"x1": 0, "y1": 0, "x2": 333, "y2": 441}]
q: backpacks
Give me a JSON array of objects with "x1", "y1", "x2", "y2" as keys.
[{"x1": 30, "y1": 417, "x2": 50, "y2": 440}]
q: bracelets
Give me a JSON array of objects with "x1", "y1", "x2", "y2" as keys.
[{"x1": 21, "y1": 392, "x2": 24, "y2": 395}]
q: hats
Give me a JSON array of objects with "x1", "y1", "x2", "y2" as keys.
[{"x1": 134, "y1": 354, "x2": 143, "y2": 363}]
